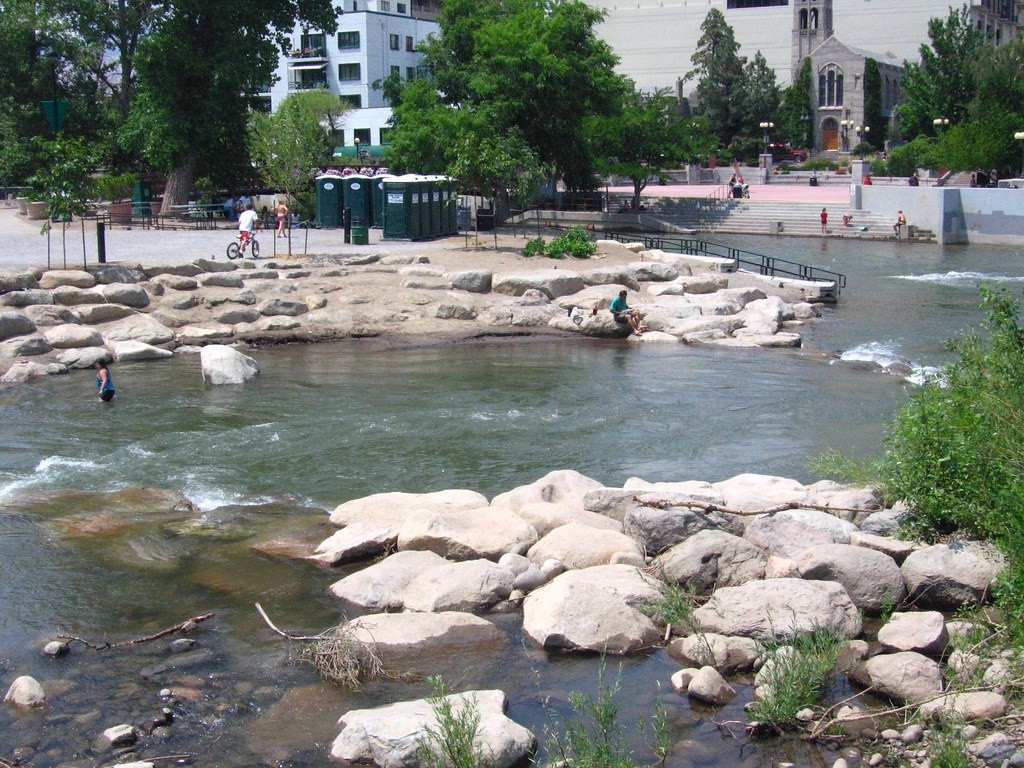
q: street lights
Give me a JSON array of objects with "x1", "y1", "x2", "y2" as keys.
[
  {"x1": 841, "y1": 120, "x2": 854, "y2": 153},
  {"x1": 355, "y1": 137, "x2": 360, "y2": 160},
  {"x1": 760, "y1": 122, "x2": 774, "y2": 154},
  {"x1": 855, "y1": 126, "x2": 870, "y2": 160}
]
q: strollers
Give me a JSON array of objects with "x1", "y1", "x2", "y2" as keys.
[{"x1": 742, "y1": 183, "x2": 751, "y2": 199}]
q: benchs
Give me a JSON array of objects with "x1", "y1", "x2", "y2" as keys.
[
  {"x1": 181, "y1": 210, "x2": 224, "y2": 221},
  {"x1": 170, "y1": 210, "x2": 224, "y2": 219}
]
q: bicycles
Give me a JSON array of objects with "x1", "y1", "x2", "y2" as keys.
[{"x1": 227, "y1": 226, "x2": 260, "y2": 259}]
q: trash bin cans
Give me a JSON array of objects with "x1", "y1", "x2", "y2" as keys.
[
  {"x1": 351, "y1": 221, "x2": 369, "y2": 245},
  {"x1": 810, "y1": 175, "x2": 817, "y2": 186},
  {"x1": 733, "y1": 183, "x2": 742, "y2": 197}
]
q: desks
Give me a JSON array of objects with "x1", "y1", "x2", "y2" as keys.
[{"x1": 170, "y1": 204, "x2": 224, "y2": 219}]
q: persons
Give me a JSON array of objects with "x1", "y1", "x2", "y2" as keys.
[
  {"x1": 94, "y1": 359, "x2": 115, "y2": 401},
  {"x1": 909, "y1": 172, "x2": 919, "y2": 186},
  {"x1": 970, "y1": 174, "x2": 977, "y2": 187},
  {"x1": 893, "y1": 211, "x2": 905, "y2": 236},
  {"x1": 272, "y1": 200, "x2": 288, "y2": 238},
  {"x1": 624, "y1": 200, "x2": 629, "y2": 211},
  {"x1": 224, "y1": 193, "x2": 251, "y2": 220},
  {"x1": 261, "y1": 206, "x2": 269, "y2": 230},
  {"x1": 238, "y1": 204, "x2": 259, "y2": 258},
  {"x1": 820, "y1": 208, "x2": 828, "y2": 233},
  {"x1": 609, "y1": 290, "x2": 647, "y2": 335},
  {"x1": 631, "y1": 197, "x2": 635, "y2": 208},
  {"x1": 863, "y1": 176, "x2": 870, "y2": 185},
  {"x1": 728, "y1": 173, "x2": 743, "y2": 197},
  {"x1": 290, "y1": 209, "x2": 300, "y2": 228},
  {"x1": 842, "y1": 214, "x2": 853, "y2": 227},
  {"x1": 206, "y1": 192, "x2": 222, "y2": 218}
]
